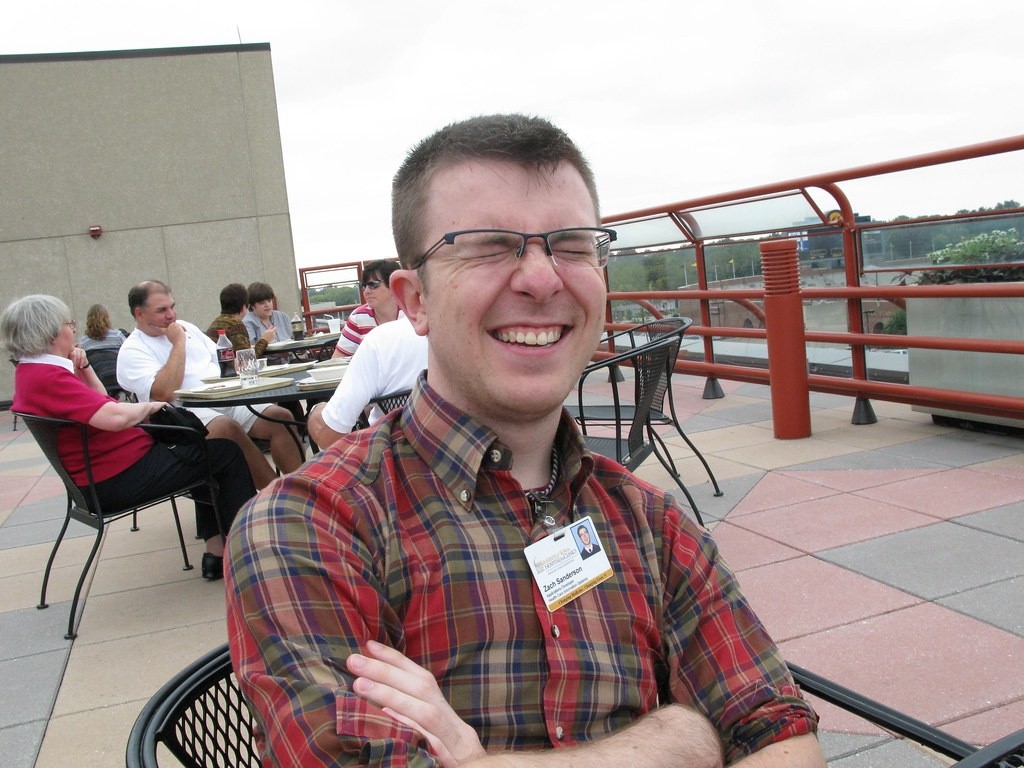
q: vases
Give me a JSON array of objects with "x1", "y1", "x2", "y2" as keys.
[{"x1": 905, "y1": 285, "x2": 1024, "y2": 437}]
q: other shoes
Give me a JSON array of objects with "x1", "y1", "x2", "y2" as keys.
[{"x1": 200, "y1": 552, "x2": 225, "y2": 579}]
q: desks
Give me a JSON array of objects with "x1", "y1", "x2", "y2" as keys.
[
  {"x1": 175, "y1": 356, "x2": 360, "y2": 480},
  {"x1": 252, "y1": 332, "x2": 342, "y2": 362}
]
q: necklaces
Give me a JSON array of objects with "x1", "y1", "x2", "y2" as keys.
[
  {"x1": 261, "y1": 317, "x2": 280, "y2": 342},
  {"x1": 524, "y1": 442, "x2": 558, "y2": 503}
]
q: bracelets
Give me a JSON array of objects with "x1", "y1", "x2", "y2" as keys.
[
  {"x1": 309, "y1": 403, "x2": 325, "y2": 414},
  {"x1": 78, "y1": 361, "x2": 91, "y2": 369}
]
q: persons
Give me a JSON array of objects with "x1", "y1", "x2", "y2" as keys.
[
  {"x1": 330, "y1": 261, "x2": 403, "y2": 416},
  {"x1": 305, "y1": 315, "x2": 428, "y2": 463},
  {"x1": 222, "y1": 113, "x2": 828, "y2": 768},
  {"x1": 79, "y1": 304, "x2": 127, "y2": 400},
  {"x1": 3, "y1": 294, "x2": 256, "y2": 578},
  {"x1": 116, "y1": 281, "x2": 305, "y2": 490},
  {"x1": 203, "y1": 284, "x2": 309, "y2": 437},
  {"x1": 241, "y1": 282, "x2": 310, "y2": 365}
]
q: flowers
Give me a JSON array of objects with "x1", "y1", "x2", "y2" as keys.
[{"x1": 911, "y1": 223, "x2": 1024, "y2": 285}]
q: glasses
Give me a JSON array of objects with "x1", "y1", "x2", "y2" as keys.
[
  {"x1": 61, "y1": 319, "x2": 77, "y2": 330},
  {"x1": 359, "y1": 279, "x2": 387, "y2": 292},
  {"x1": 412, "y1": 226, "x2": 620, "y2": 273},
  {"x1": 247, "y1": 304, "x2": 250, "y2": 309}
]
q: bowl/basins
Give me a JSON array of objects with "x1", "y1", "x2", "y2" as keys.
[
  {"x1": 306, "y1": 366, "x2": 348, "y2": 382},
  {"x1": 257, "y1": 358, "x2": 268, "y2": 372}
]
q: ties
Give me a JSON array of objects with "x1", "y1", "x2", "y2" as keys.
[{"x1": 589, "y1": 549, "x2": 592, "y2": 552}]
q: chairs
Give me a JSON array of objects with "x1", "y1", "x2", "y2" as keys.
[
  {"x1": 572, "y1": 338, "x2": 703, "y2": 524},
  {"x1": 124, "y1": 617, "x2": 998, "y2": 768},
  {"x1": 8, "y1": 355, "x2": 21, "y2": 429},
  {"x1": 596, "y1": 316, "x2": 723, "y2": 497},
  {"x1": 85, "y1": 346, "x2": 134, "y2": 403},
  {"x1": 12, "y1": 409, "x2": 229, "y2": 639},
  {"x1": 133, "y1": 372, "x2": 283, "y2": 470},
  {"x1": 352, "y1": 388, "x2": 418, "y2": 433}
]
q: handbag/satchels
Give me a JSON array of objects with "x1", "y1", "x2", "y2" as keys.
[{"x1": 150, "y1": 404, "x2": 209, "y2": 448}]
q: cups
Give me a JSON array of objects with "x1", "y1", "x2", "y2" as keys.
[
  {"x1": 236, "y1": 349, "x2": 259, "y2": 385},
  {"x1": 327, "y1": 318, "x2": 341, "y2": 334}
]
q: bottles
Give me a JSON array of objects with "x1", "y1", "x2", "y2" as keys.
[
  {"x1": 216, "y1": 329, "x2": 236, "y2": 377},
  {"x1": 291, "y1": 312, "x2": 304, "y2": 341}
]
